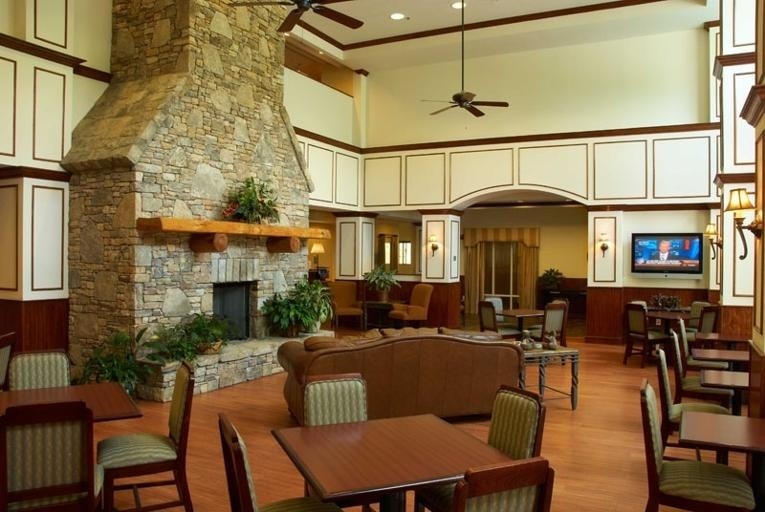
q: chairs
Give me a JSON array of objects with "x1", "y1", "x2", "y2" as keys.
[
  {"x1": 385, "y1": 281, "x2": 434, "y2": 327},
  {"x1": 216, "y1": 407, "x2": 345, "y2": 512},
  {"x1": 622, "y1": 291, "x2": 765, "y2": 511},
  {"x1": 328, "y1": 278, "x2": 362, "y2": 328},
  {"x1": 7, "y1": 347, "x2": 71, "y2": 391},
  {"x1": 2, "y1": 400, "x2": 105, "y2": 511},
  {"x1": 1, "y1": 330, "x2": 18, "y2": 387},
  {"x1": 421, "y1": 383, "x2": 556, "y2": 508},
  {"x1": 302, "y1": 370, "x2": 377, "y2": 512},
  {"x1": 96, "y1": 359, "x2": 200, "y2": 511},
  {"x1": 475, "y1": 293, "x2": 569, "y2": 344}
]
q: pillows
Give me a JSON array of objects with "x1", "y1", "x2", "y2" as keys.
[{"x1": 341, "y1": 326, "x2": 503, "y2": 343}]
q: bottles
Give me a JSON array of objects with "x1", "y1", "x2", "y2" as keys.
[
  {"x1": 543, "y1": 329, "x2": 557, "y2": 350},
  {"x1": 521, "y1": 330, "x2": 533, "y2": 350}
]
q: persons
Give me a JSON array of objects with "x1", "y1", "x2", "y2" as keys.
[{"x1": 652, "y1": 239, "x2": 679, "y2": 260}]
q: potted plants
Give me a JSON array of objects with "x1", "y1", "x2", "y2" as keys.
[
  {"x1": 188, "y1": 311, "x2": 236, "y2": 354},
  {"x1": 361, "y1": 265, "x2": 401, "y2": 302},
  {"x1": 262, "y1": 292, "x2": 301, "y2": 339},
  {"x1": 223, "y1": 176, "x2": 281, "y2": 223},
  {"x1": 294, "y1": 273, "x2": 334, "y2": 334}
]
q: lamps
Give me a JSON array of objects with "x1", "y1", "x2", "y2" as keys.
[
  {"x1": 429, "y1": 235, "x2": 439, "y2": 257},
  {"x1": 703, "y1": 222, "x2": 719, "y2": 261},
  {"x1": 723, "y1": 188, "x2": 761, "y2": 260},
  {"x1": 311, "y1": 242, "x2": 326, "y2": 268},
  {"x1": 599, "y1": 234, "x2": 609, "y2": 258}
]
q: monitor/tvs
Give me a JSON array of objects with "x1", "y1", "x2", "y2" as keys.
[{"x1": 631, "y1": 232, "x2": 704, "y2": 280}]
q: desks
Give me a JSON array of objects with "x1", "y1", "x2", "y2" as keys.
[
  {"x1": 0, "y1": 381, "x2": 146, "y2": 425},
  {"x1": 273, "y1": 413, "x2": 511, "y2": 500},
  {"x1": 503, "y1": 337, "x2": 581, "y2": 411}
]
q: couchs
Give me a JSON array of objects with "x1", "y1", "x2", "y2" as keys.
[{"x1": 274, "y1": 326, "x2": 526, "y2": 421}]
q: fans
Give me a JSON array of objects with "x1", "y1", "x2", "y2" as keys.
[
  {"x1": 225, "y1": 0, "x2": 365, "y2": 33},
  {"x1": 421, "y1": 0, "x2": 509, "y2": 118}
]
